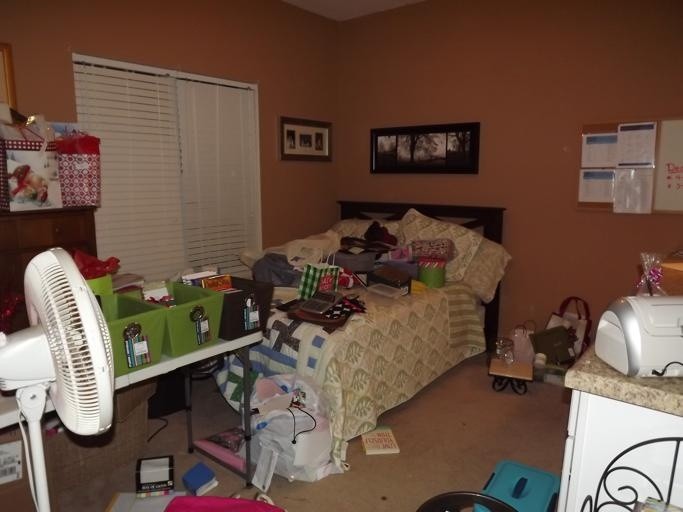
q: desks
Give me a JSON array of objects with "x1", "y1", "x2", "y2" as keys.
[{"x1": 0, "y1": 330, "x2": 264, "y2": 489}]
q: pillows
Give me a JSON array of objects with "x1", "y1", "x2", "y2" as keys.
[
  {"x1": 332, "y1": 218, "x2": 399, "y2": 240},
  {"x1": 463, "y1": 237, "x2": 511, "y2": 303},
  {"x1": 394, "y1": 208, "x2": 483, "y2": 282}
]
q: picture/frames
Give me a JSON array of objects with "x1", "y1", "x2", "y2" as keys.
[
  {"x1": 369, "y1": 120, "x2": 481, "y2": 177},
  {"x1": 279, "y1": 115, "x2": 333, "y2": 164}
]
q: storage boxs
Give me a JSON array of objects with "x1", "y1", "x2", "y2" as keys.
[
  {"x1": 474, "y1": 459, "x2": 560, "y2": 512},
  {"x1": 84, "y1": 272, "x2": 275, "y2": 377}
]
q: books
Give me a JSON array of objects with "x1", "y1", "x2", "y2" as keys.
[
  {"x1": 360, "y1": 424, "x2": 401, "y2": 455},
  {"x1": 179, "y1": 269, "x2": 243, "y2": 295},
  {"x1": 181, "y1": 462, "x2": 218, "y2": 496}
]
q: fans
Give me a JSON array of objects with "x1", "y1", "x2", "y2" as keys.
[{"x1": 0, "y1": 246, "x2": 118, "y2": 507}]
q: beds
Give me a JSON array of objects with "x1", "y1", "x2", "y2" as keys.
[{"x1": 228, "y1": 201, "x2": 505, "y2": 441}]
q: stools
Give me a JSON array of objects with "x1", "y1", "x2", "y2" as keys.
[{"x1": 486, "y1": 357, "x2": 534, "y2": 394}]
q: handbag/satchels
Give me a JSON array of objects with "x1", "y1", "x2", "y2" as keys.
[
  {"x1": 503, "y1": 318, "x2": 538, "y2": 363},
  {"x1": 297, "y1": 248, "x2": 339, "y2": 300},
  {"x1": 0, "y1": 122, "x2": 63, "y2": 213},
  {"x1": 55, "y1": 127, "x2": 100, "y2": 209},
  {"x1": 544, "y1": 295, "x2": 591, "y2": 363}
]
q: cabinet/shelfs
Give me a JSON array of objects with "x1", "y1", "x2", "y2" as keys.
[
  {"x1": 556, "y1": 341, "x2": 683, "y2": 511},
  {"x1": 2, "y1": 207, "x2": 98, "y2": 333}
]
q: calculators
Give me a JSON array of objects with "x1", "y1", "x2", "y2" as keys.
[{"x1": 300, "y1": 290, "x2": 336, "y2": 315}]
